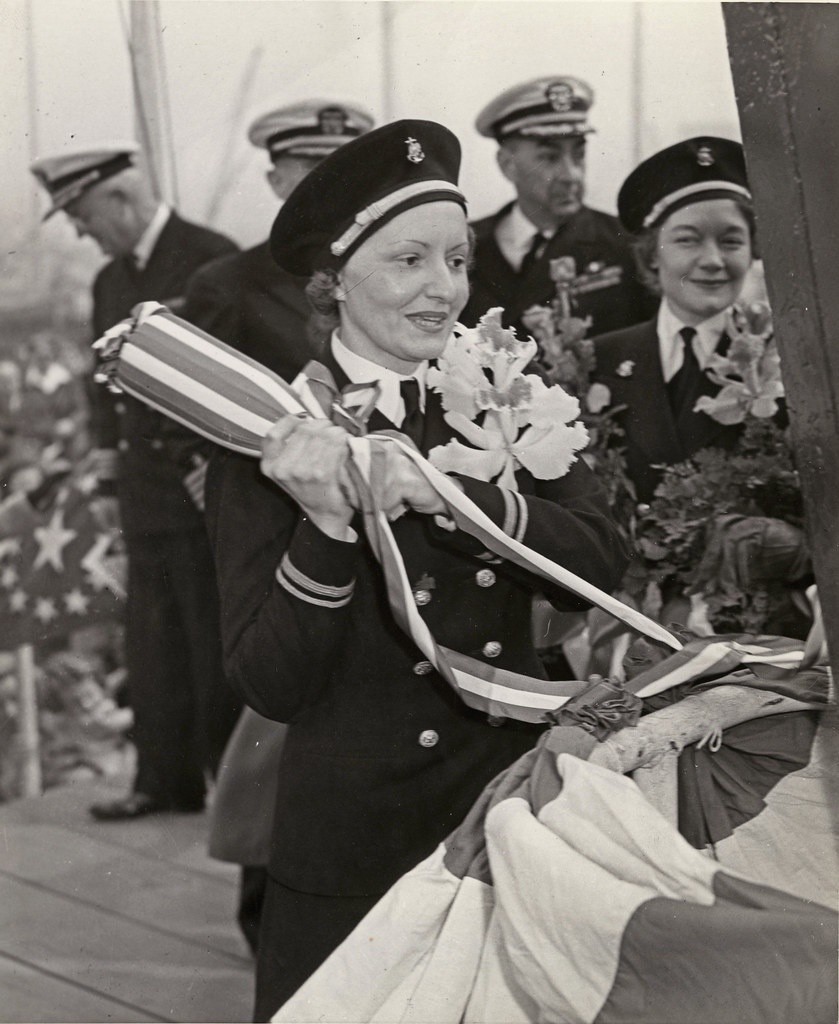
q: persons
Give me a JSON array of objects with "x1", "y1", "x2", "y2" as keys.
[
  {"x1": 27, "y1": 143, "x2": 247, "y2": 821},
  {"x1": 204, "y1": 119, "x2": 629, "y2": 1024},
  {"x1": 186, "y1": 76, "x2": 791, "y2": 956}
]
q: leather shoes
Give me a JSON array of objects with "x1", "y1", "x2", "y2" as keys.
[{"x1": 90, "y1": 792, "x2": 206, "y2": 821}]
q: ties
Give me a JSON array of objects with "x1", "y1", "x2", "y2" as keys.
[
  {"x1": 399, "y1": 379, "x2": 425, "y2": 452},
  {"x1": 668, "y1": 326, "x2": 701, "y2": 422},
  {"x1": 520, "y1": 232, "x2": 551, "y2": 274}
]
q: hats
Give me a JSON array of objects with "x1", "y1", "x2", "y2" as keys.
[
  {"x1": 269, "y1": 120, "x2": 469, "y2": 281},
  {"x1": 28, "y1": 149, "x2": 134, "y2": 223},
  {"x1": 248, "y1": 99, "x2": 375, "y2": 163},
  {"x1": 476, "y1": 76, "x2": 596, "y2": 146},
  {"x1": 617, "y1": 137, "x2": 753, "y2": 239}
]
